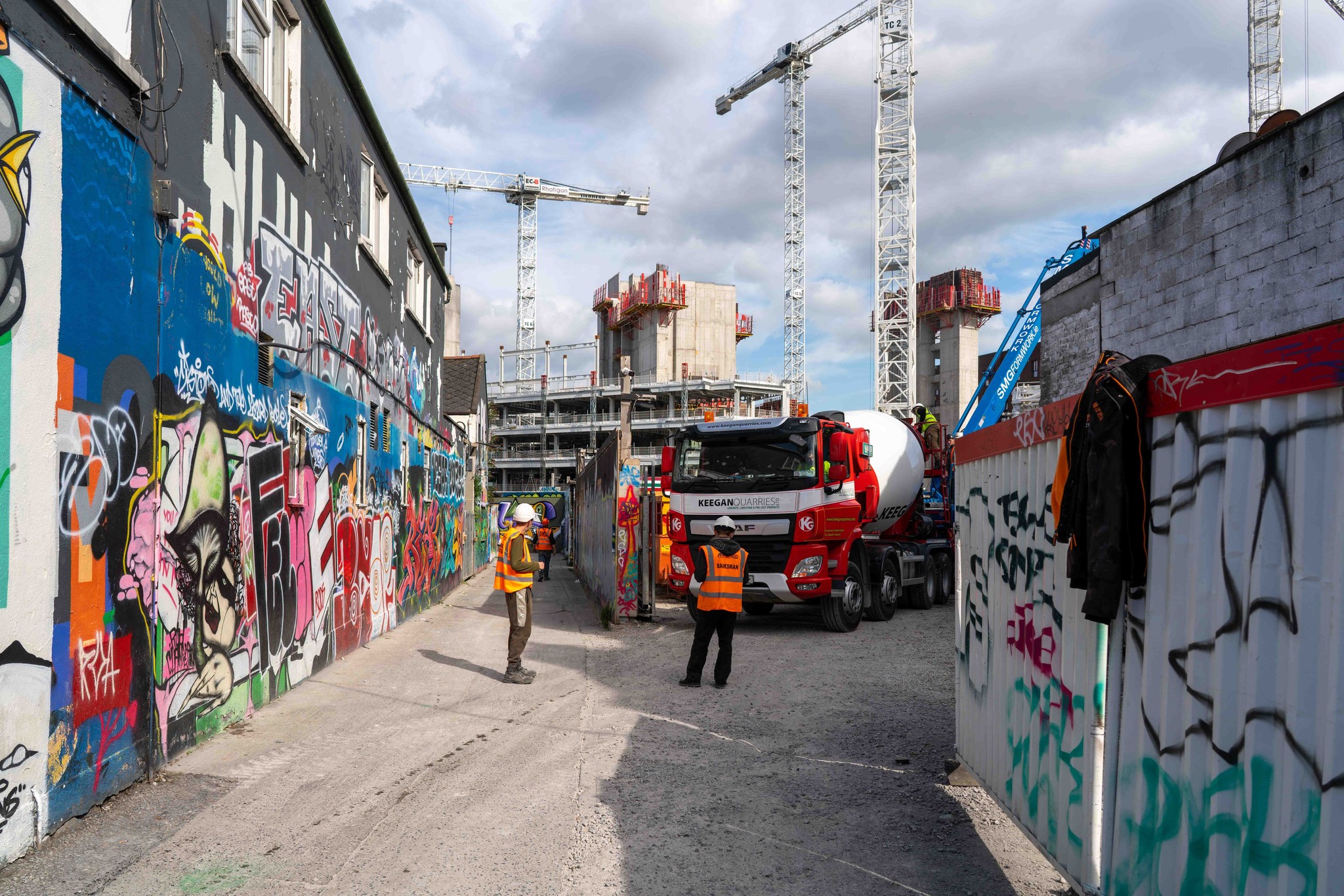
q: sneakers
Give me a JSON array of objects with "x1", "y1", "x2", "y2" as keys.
[
  {"x1": 679, "y1": 678, "x2": 701, "y2": 687},
  {"x1": 715, "y1": 682, "x2": 727, "y2": 689}
]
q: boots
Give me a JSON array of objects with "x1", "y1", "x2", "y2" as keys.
[
  {"x1": 517, "y1": 658, "x2": 537, "y2": 677},
  {"x1": 503, "y1": 664, "x2": 534, "y2": 684}
]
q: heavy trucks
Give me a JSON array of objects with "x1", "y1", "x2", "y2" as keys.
[{"x1": 660, "y1": 408, "x2": 955, "y2": 634}]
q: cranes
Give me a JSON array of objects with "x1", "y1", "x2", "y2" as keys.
[
  {"x1": 395, "y1": 161, "x2": 649, "y2": 389},
  {"x1": 710, "y1": 0, "x2": 881, "y2": 415},
  {"x1": 1247, "y1": 0, "x2": 1344, "y2": 135}
]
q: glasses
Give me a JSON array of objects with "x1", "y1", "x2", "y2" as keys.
[{"x1": 914, "y1": 408, "x2": 917, "y2": 413}]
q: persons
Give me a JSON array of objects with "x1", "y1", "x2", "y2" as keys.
[
  {"x1": 533, "y1": 518, "x2": 556, "y2": 582},
  {"x1": 493, "y1": 503, "x2": 545, "y2": 685},
  {"x1": 943, "y1": 434, "x2": 955, "y2": 505},
  {"x1": 679, "y1": 516, "x2": 749, "y2": 689},
  {"x1": 911, "y1": 403, "x2": 946, "y2": 470}
]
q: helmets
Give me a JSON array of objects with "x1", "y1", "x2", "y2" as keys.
[
  {"x1": 947, "y1": 434, "x2": 955, "y2": 439},
  {"x1": 542, "y1": 518, "x2": 549, "y2": 526},
  {"x1": 713, "y1": 516, "x2": 736, "y2": 530},
  {"x1": 911, "y1": 403, "x2": 925, "y2": 414},
  {"x1": 513, "y1": 503, "x2": 536, "y2": 522}
]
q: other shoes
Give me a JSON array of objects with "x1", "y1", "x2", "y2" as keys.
[
  {"x1": 950, "y1": 500, "x2": 954, "y2": 504},
  {"x1": 544, "y1": 577, "x2": 551, "y2": 580},
  {"x1": 933, "y1": 460, "x2": 946, "y2": 469},
  {"x1": 535, "y1": 575, "x2": 543, "y2": 582}
]
